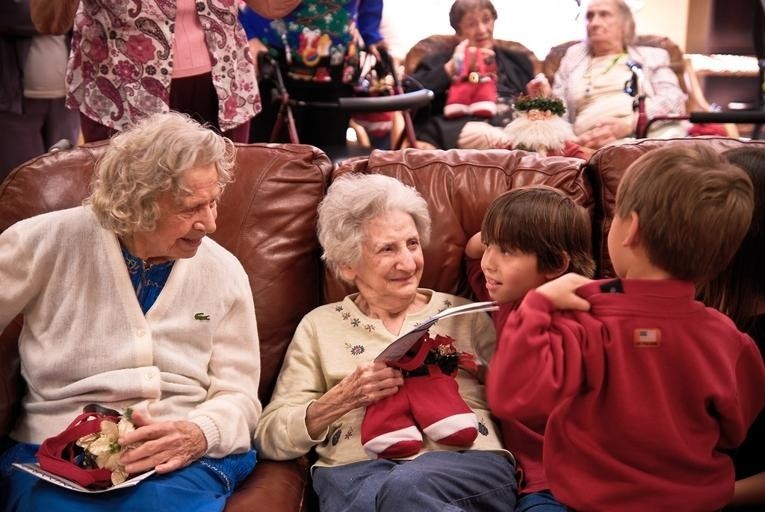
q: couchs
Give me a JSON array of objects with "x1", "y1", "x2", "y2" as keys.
[{"x1": 0, "y1": 129, "x2": 764, "y2": 507}]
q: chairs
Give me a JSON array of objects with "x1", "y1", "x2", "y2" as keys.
[
  {"x1": 543, "y1": 37, "x2": 740, "y2": 138},
  {"x1": 406, "y1": 34, "x2": 540, "y2": 149}
]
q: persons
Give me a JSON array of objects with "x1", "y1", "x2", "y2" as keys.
[
  {"x1": 712, "y1": 142, "x2": 765, "y2": 511},
  {"x1": 0, "y1": 108, "x2": 265, "y2": 512},
  {"x1": 463, "y1": 182, "x2": 598, "y2": 511},
  {"x1": 403, "y1": 0, "x2": 536, "y2": 158},
  {"x1": 0, "y1": 1, "x2": 416, "y2": 185},
  {"x1": 527, "y1": 0, "x2": 698, "y2": 152},
  {"x1": 485, "y1": 138, "x2": 764, "y2": 512},
  {"x1": 250, "y1": 168, "x2": 522, "y2": 512}
]
